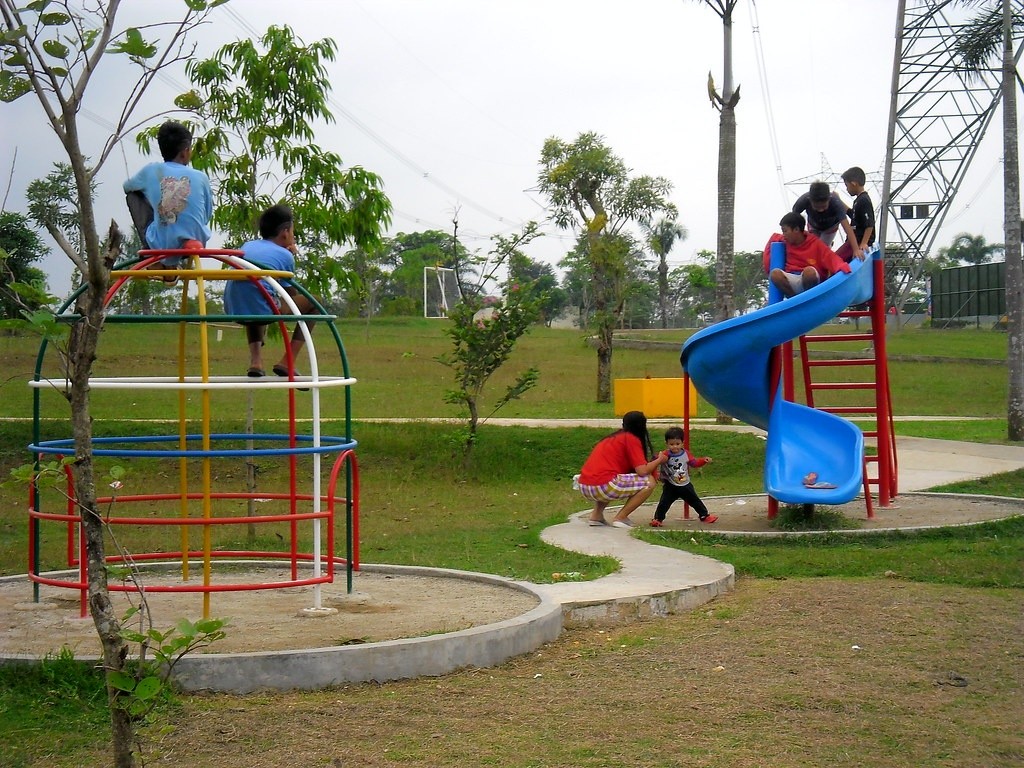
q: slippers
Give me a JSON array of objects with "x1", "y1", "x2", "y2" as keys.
[
  {"x1": 273, "y1": 364, "x2": 310, "y2": 391},
  {"x1": 248, "y1": 367, "x2": 265, "y2": 376}
]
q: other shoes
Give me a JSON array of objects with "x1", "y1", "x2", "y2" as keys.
[
  {"x1": 705, "y1": 515, "x2": 719, "y2": 523},
  {"x1": 650, "y1": 520, "x2": 664, "y2": 526},
  {"x1": 588, "y1": 519, "x2": 612, "y2": 527},
  {"x1": 144, "y1": 263, "x2": 181, "y2": 286},
  {"x1": 612, "y1": 517, "x2": 640, "y2": 529}
]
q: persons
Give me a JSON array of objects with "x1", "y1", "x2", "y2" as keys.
[
  {"x1": 121, "y1": 119, "x2": 214, "y2": 289},
  {"x1": 791, "y1": 180, "x2": 866, "y2": 277},
  {"x1": 579, "y1": 410, "x2": 668, "y2": 531},
  {"x1": 648, "y1": 425, "x2": 719, "y2": 528},
  {"x1": 222, "y1": 202, "x2": 325, "y2": 392},
  {"x1": 833, "y1": 165, "x2": 876, "y2": 266},
  {"x1": 761, "y1": 211, "x2": 853, "y2": 299},
  {"x1": 887, "y1": 304, "x2": 896, "y2": 314}
]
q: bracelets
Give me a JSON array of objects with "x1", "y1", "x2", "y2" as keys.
[{"x1": 289, "y1": 249, "x2": 295, "y2": 256}]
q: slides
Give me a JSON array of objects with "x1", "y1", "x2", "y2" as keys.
[{"x1": 678, "y1": 239, "x2": 875, "y2": 504}]
